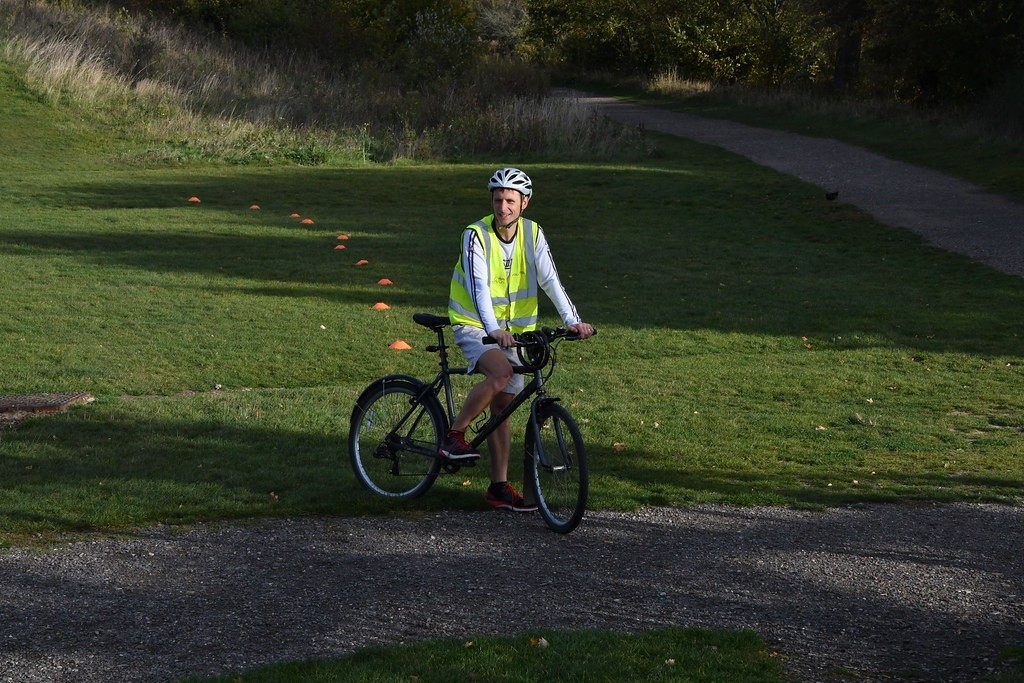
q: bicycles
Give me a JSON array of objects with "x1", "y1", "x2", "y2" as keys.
[{"x1": 348, "y1": 313, "x2": 598, "y2": 534}]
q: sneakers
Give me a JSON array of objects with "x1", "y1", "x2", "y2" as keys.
[
  {"x1": 483, "y1": 480, "x2": 539, "y2": 512},
  {"x1": 436, "y1": 429, "x2": 481, "y2": 461}
]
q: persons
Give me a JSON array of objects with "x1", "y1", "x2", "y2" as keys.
[{"x1": 438, "y1": 168, "x2": 594, "y2": 512}]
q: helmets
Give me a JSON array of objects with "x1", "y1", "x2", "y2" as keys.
[{"x1": 487, "y1": 167, "x2": 533, "y2": 201}]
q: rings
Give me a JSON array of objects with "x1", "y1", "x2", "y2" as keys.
[{"x1": 588, "y1": 328, "x2": 590, "y2": 332}]
